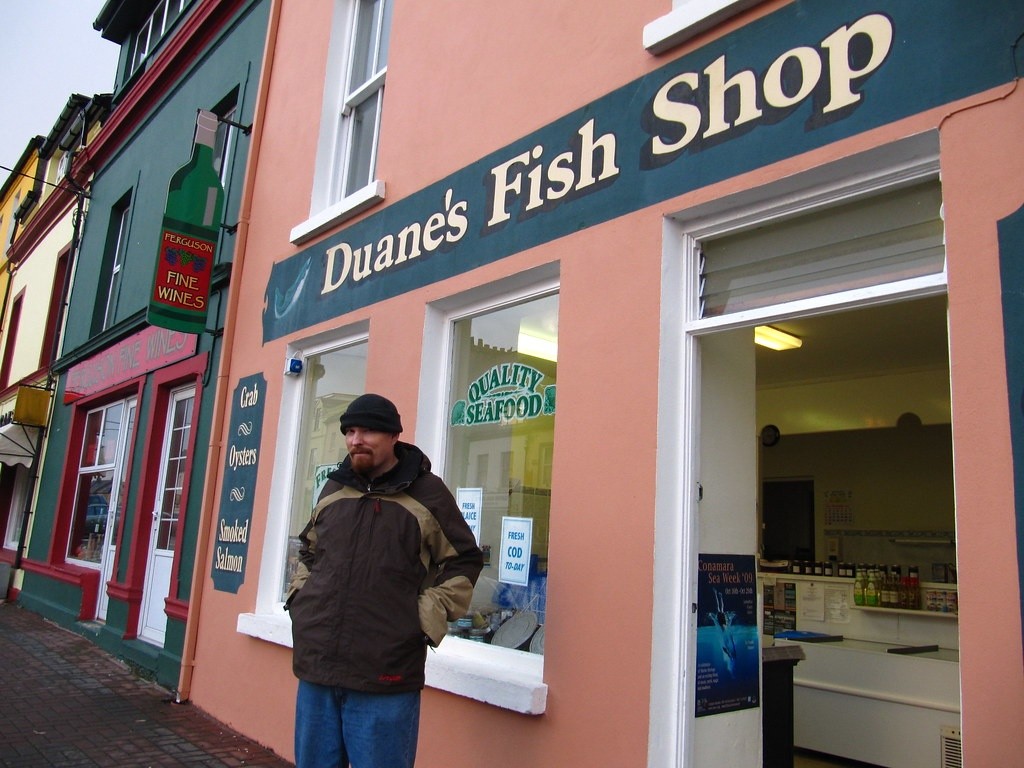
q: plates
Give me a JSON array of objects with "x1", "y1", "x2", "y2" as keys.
[
  {"x1": 491, "y1": 611, "x2": 538, "y2": 649},
  {"x1": 529, "y1": 624, "x2": 544, "y2": 655}
]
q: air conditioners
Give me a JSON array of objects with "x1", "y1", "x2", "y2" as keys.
[{"x1": 56, "y1": 152, "x2": 68, "y2": 185}]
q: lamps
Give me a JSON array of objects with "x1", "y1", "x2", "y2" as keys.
[{"x1": 754, "y1": 325, "x2": 804, "y2": 360}]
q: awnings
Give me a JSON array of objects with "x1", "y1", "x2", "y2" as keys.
[{"x1": 0, "y1": 421, "x2": 39, "y2": 468}]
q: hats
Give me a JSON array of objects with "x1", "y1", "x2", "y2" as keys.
[{"x1": 339, "y1": 393, "x2": 404, "y2": 435}]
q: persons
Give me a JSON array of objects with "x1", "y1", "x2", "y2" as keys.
[{"x1": 283, "y1": 393, "x2": 485, "y2": 768}]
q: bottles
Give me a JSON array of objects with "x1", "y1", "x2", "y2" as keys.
[{"x1": 792, "y1": 560, "x2": 919, "y2": 608}]
